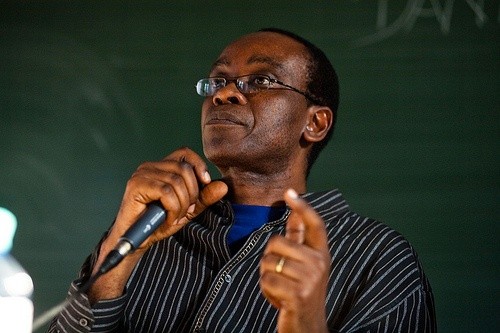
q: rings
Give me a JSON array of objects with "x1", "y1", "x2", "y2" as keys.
[{"x1": 277, "y1": 255, "x2": 286, "y2": 274}]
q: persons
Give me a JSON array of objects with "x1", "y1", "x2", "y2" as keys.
[{"x1": 46, "y1": 26, "x2": 436, "y2": 333}]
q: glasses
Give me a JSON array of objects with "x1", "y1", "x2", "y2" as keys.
[{"x1": 194, "y1": 74, "x2": 322, "y2": 106}]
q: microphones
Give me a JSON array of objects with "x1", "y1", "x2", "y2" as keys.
[{"x1": 98, "y1": 160, "x2": 206, "y2": 273}]
